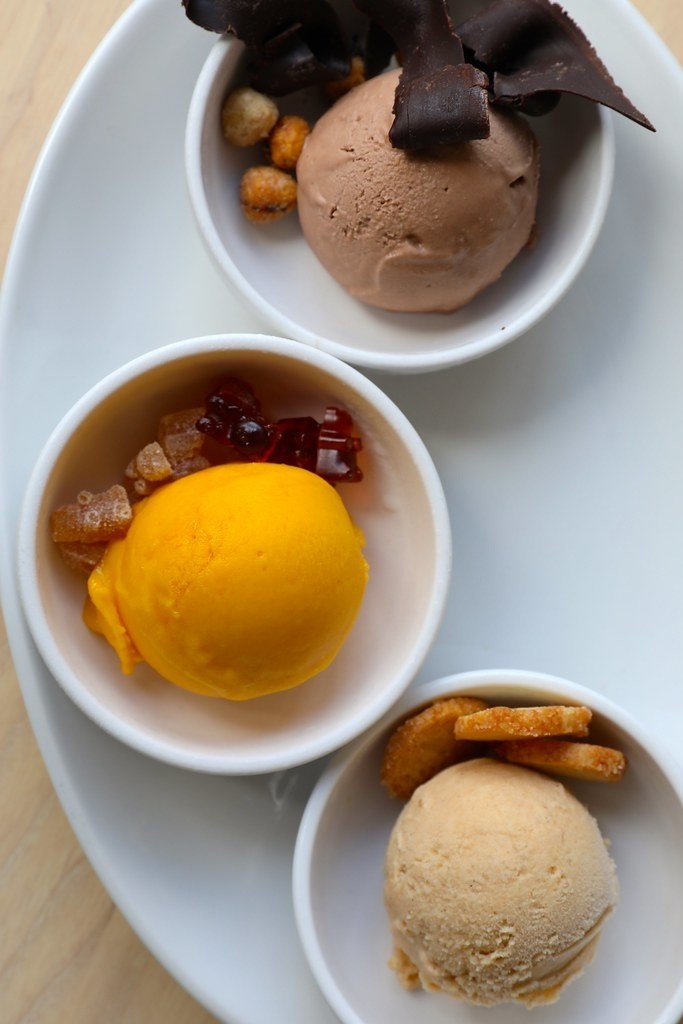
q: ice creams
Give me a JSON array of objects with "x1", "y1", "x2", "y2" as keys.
[
  {"x1": 82, "y1": 460, "x2": 370, "y2": 701},
  {"x1": 294, "y1": 65, "x2": 543, "y2": 310},
  {"x1": 383, "y1": 758, "x2": 621, "y2": 1009}
]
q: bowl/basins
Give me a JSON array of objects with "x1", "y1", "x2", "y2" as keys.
[
  {"x1": 186, "y1": 33, "x2": 616, "y2": 372},
  {"x1": 291, "y1": 669, "x2": 683, "y2": 1024},
  {"x1": 19, "y1": 334, "x2": 452, "y2": 775}
]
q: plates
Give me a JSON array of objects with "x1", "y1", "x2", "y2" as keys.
[{"x1": 0, "y1": 0, "x2": 683, "y2": 1023}]
